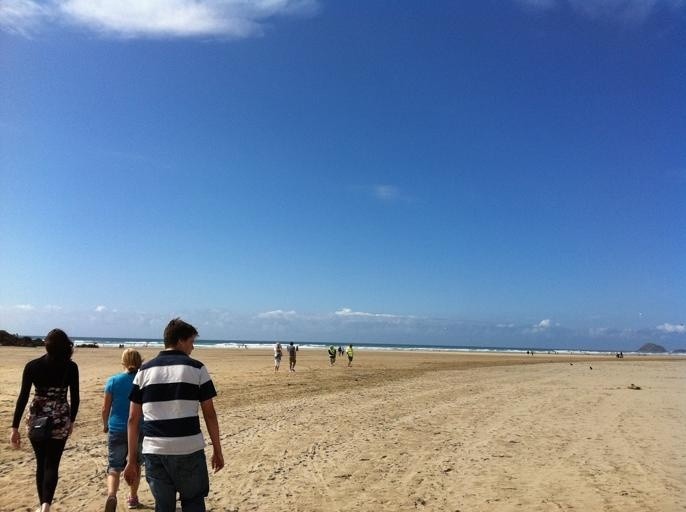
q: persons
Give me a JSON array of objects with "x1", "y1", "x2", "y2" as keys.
[
  {"x1": 9, "y1": 327, "x2": 82, "y2": 512},
  {"x1": 286, "y1": 341, "x2": 295, "y2": 372},
  {"x1": 327, "y1": 343, "x2": 336, "y2": 367},
  {"x1": 100, "y1": 348, "x2": 149, "y2": 511},
  {"x1": 272, "y1": 340, "x2": 283, "y2": 374},
  {"x1": 340, "y1": 345, "x2": 345, "y2": 355},
  {"x1": 346, "y1": 343, "x2": 355, "y2": 367},
  {"x1": 337, "y1": 346, "x2": 340, "y2": 355},
  {"x1": 122, "y1": 314, "x2": 224, "y2": 511}
]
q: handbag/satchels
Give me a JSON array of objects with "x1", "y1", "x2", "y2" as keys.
[{"x1": 31, "y1": 416, "x2": 52, "y2": 439}]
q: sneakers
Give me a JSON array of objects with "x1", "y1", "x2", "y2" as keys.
[
  {"x1": 105, "y1": 495, "x2": 117, "y2": 512},
  {"x1": 127, "y1": 495, "x2": 140, "y2": 509}
]
